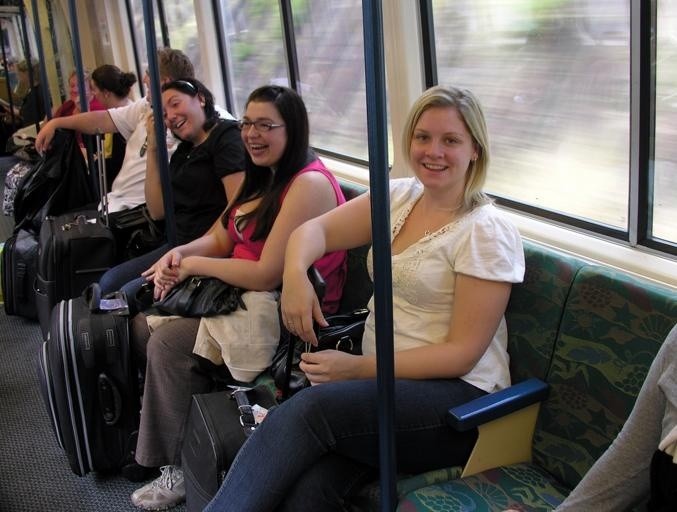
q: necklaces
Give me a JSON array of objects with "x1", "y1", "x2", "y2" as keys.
[{"x1": 424, "y1": 227, "x2": 431, "y2": 237}]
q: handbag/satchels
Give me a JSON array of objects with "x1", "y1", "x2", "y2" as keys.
[
  {"x1": 273, "y1": 309, "x2": 368, "y2": 397},
  {"x1": 155, "y1": 274, "x2": 247, "y2": 316}
]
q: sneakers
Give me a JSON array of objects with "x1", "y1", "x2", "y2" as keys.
[{"x1": 132, "y1": 464, "x2": 185, "y2": 510}]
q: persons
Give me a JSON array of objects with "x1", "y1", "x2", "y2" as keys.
[
  {"x1": 200, "y1": 84, "x2": 526, "y2": 511},
  {"x1": 129, "y1": 85, "x2": 351, "y2": 511},
  {"x1": 96, "y1": 78, "x2": 246, "y2": 312},
  {"x1": 550, "y1": 320, "x2": 676, "y2": 512}
]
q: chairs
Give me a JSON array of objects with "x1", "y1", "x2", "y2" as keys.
[{"x1": 362, "y1": 236, "x2": 677, "y2": 510}]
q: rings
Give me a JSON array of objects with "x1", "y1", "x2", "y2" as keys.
[{"x1": 286, "y1": 319, "x2": 295, "y2": 324}]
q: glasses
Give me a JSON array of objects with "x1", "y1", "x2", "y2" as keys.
[{"x1": 238, "y1": 115, "x2": 284, "y2": 134}]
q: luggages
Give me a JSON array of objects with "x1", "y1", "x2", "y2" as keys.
[
  {"x1": 180, "y1": 385, "x2": 278, "y2": 511},
  {"x1": 2, "y1": 129, "x2": 140, "y2": 478}
]
